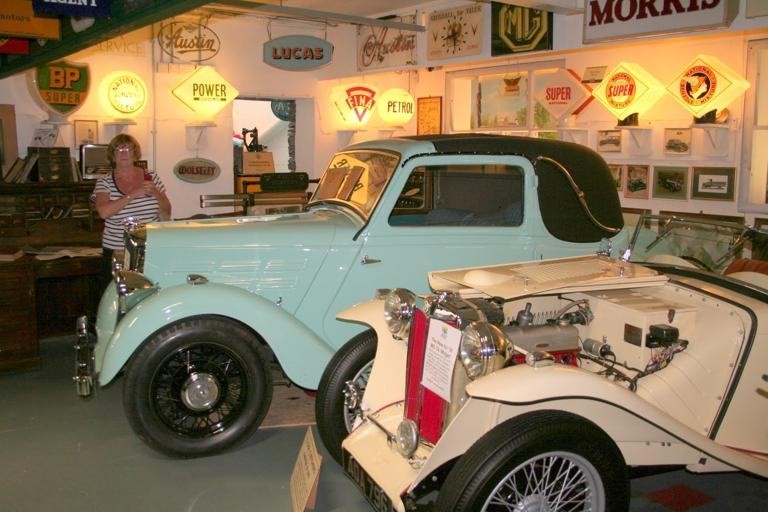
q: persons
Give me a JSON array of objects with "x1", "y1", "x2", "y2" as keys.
[{"x1": 90, "y1": 133, "x2": 172, "y2": 338}]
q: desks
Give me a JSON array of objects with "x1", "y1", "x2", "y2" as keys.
[{"x1": 1, "y1": 243, "x2": 117, "y2": 376}]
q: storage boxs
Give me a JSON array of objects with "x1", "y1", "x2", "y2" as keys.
[{"x1": 26, "y1": 146, "x2": 70, "y2": 182}]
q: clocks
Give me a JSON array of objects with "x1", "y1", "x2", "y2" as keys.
[{"x1": 426, "y1": 1, "x2": 485, "y2": 61}]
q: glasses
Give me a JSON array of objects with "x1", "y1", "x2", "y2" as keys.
[{"x1": 114, "y1": 146, "x2": 135, "y2": 153}]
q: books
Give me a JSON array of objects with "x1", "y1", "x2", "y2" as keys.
[
  {"x1": 1, "y1": 205, "x2": 104, "y2": 261},
  {"x1": 2, "y1": 153, "x2": 39, "y2": 184},
  {"x1": 71, "y1": 157, "x2": 84, "y2": 183}
]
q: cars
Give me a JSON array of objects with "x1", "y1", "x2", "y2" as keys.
[
  {"x1": 600, "y1": 136, "x2": 621, "y2": 146},
  {"x1": 629, "y1": 179, "x2": 646, "y2": 192},
  {"x1": 666, "y1": 138, "x2": 688, "y2": 152},
  {"x1": 659, "y1": 175, "x2": 681, "y2": 192}
]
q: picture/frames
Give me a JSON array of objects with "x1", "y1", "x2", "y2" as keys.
[
  {"x1": 73, "y1": 118, "x2": 99, "y2": 149},
  {"x1": 658, "y1": 209, "x2": 743, "y2": 269},
  {"x1": 622, "y1": 162, "x2": 649, "y2": 201},
  {"x1": 582, "y1": 1, "x2": 737, "y2": 46},
  {"x1": 608, "y1": 164, "x2": 624, "y2": 193},
  {"x1": 752, "y1": 217, "x2": 767, "y2": 263},
  {"x1": 416, "y1": 95, "x2": 442, "y2": 134},
  {"x1": 595, "y1": 129, "x2": 623, "y2": 154},
  {"x1": 80, "y1": 145, "x2": 114, "y2": 182},
  {"x1": 621, "y1": 208, "x2": 652, "y2": 234},
  {"x1": 688, "y1": 165, "x2": 736, "y2": 202},
  {"x1": 649, "y1": 163, "x2": 688, "y2": 201},
  {"x1": 663, "y1": 124, "x2": 692, "y2": 155}
]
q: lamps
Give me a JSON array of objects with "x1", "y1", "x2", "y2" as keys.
[
  {"x1": 329, "y1": 79, "x2": 377, "y2": 150},
  {"x1": 168, "y1": 65, "x2": 240, "y2": 151},
  {"x1": 27, "y1": 58, "x2": 92, "y2": 145},
  {"x1": 534, "y1": 67, "x2": 590, "y2": 146},
  {"x1": 589, "y1": 61, "x2": 666, "y2": 159},
  {"x1": 663, "y1": 52, "x2": 751, "y2": 157},
  {"x1": 376, "y1": 86, "x2": 415, "y2": 136},
  {"x1": 99, "y1": 67, "x2": 149, "y2": 135}
]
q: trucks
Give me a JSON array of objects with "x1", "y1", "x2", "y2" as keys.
[{"x1": 702, "y1": 178, "x2": 727, "y2": 190}]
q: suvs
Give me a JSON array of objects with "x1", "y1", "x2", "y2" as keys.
[
  {"x1": 315, "y1": 208, "x2": 768, "y2": 511},
  {"x1": 73, "y1": 134, "x2": 673, "y2": 459}
]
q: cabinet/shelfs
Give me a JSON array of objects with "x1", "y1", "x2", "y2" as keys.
[{"x1": 0, "y1": 182, "x2": 115, "y2": 248}]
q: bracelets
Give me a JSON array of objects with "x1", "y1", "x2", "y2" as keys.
[{"x1": 125, "y1": 195, "x2": 133, "y2": 202}]
